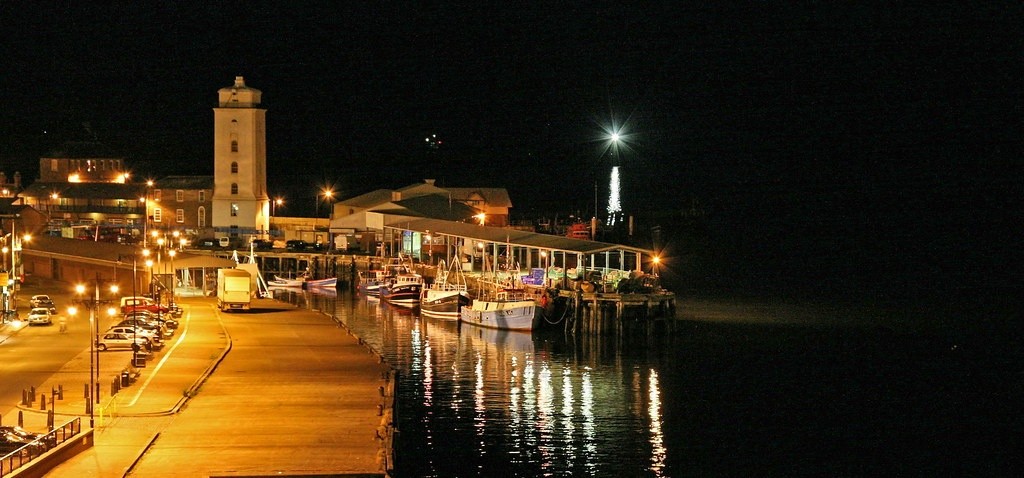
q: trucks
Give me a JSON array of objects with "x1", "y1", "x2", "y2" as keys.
[{"x1": 216, "y1": 268, "x2": 252, "y2": 312}]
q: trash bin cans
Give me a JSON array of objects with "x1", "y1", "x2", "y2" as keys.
[
  {"x1": 121, "y1": 372, "x2": 130, "y2": 387},
  {"x1": 153, "y1": 336, "x2": 160, "y2": 349}
]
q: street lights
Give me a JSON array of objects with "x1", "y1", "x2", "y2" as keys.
[
  {"x1": 157, "y1": 237, "x2": 189, "y2": 311},
  {"x1": 117, "y1": 249, "x2": 150, "y2": 366},
  {"x1": 67, "y1": 298, "x2": 116, "y2": 430},
  {"x1": 322, "y1": 189, "x2": 334, "y2": 249},
  {"x1": 75, "y1": 272, "x2": 120, "y2": 405},
  {"x1": 271, "y1": 198, "x2": 284, "y2": 224},
  {"x1": 151, "y1": 230, "x2": 180, "y2": 293}
]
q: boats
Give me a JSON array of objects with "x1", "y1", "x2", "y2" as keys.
[
  {"x1": 380, "y1": 252, "x2": 425, "y2": 309},
  {"x1": 267, "y1": 279, "x2": 302, "y2": 288},
  {"x1": 460, "y1": 243, "x2": 546, "y2": 332},
  {"x1": 355, "y1": 266, "x2": 390, "y2": 298},
  {"x1": 417, "y1": 247, "x2": 471, "y2": 319},
  {"x1": 273, "y1": 273, "x2": 338, "y2": 287}
]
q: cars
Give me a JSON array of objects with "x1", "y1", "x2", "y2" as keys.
[
  {"x1": 0, "y1": 425, "x2": 56, "y2": 450},
  {"x1": 93, "y1": 296, "x2": 169, "y2": 350},
  {"x1": 27, "y1": 306, "x2": 52, "y2": 326},
  {"x1": 0, "y1": 429, "x2": 47, "y2": 459},
  {"x1": 29, "y1": 294, "x2": 56, "y2": 314}
]
